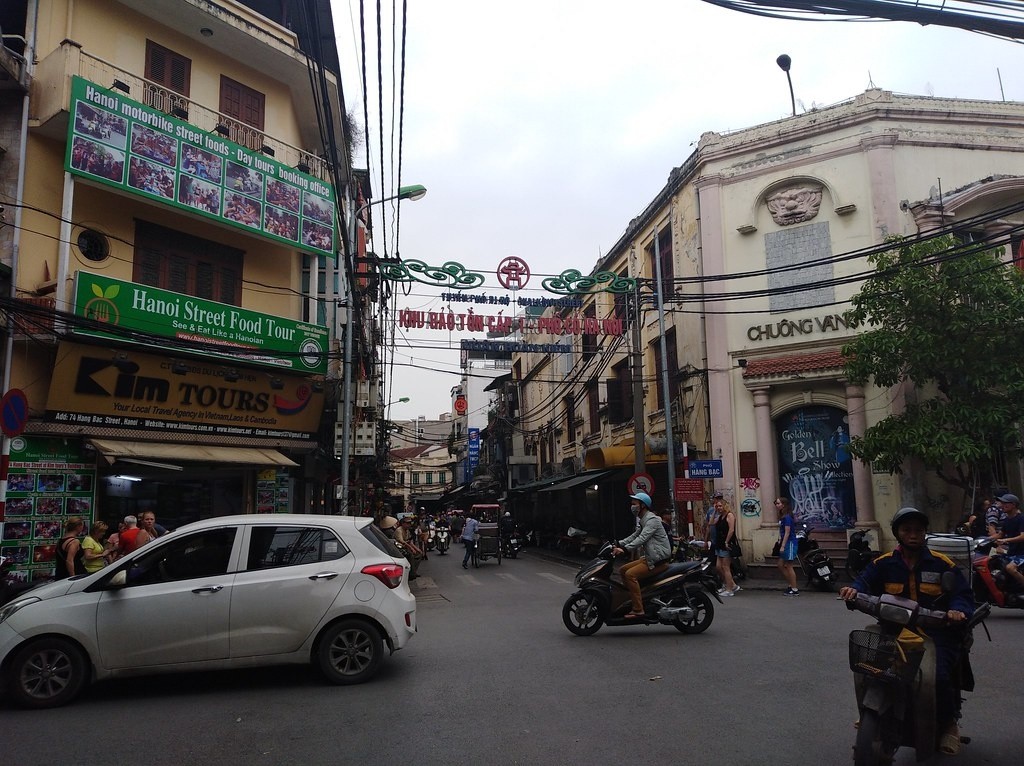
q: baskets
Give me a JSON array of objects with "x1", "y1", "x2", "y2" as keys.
[{"x1": 849, "y1": 630, "x2": 926, "y2": 687}]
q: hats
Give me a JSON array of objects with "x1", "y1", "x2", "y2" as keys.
[
  {"x1": 420, "y1": 507, "x2": 425, "y2": 510},
  {"x1": 379, "y1": 516, "x2": 398, "y2": 528},
  {"x1": 714, "y1": 492, "x2": 723, "y2": 498},
  {"x1": 997, "y1": 494, "x2": 1020, "y2": 508},
  {"x1": 403, "y1": 517, "x2": 412, "y2": 522}
]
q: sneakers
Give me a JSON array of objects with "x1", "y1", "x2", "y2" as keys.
[
  {"x1": 732, "y1": 585, "x2": 740, "y2": 592},
  {"x1": 784, "y1": 587, "x2": 799, "y2": 596},
  {"x1": 719, "y1": 591, "x2": 735, "y2": 597}
]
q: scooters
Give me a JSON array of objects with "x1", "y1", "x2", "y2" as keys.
[
  {"x1": 504, "y1": 525, "x2": 522, "y2": 557},
  {"x1": 432, "y1": 527, "x2": 450, "y2": 555},
  {"x1": 795, "y1": 524, "x2": 835, "y2": 592},
  {"x1": 844, "y1": 528, "x2": 884, "y2": 580}
]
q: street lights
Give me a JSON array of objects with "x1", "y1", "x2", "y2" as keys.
[{"x1": 339, "y1": 183, "x2": 425, "y2": 509}]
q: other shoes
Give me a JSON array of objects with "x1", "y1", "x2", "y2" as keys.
[
  {"x1": 463, "y1": 563, "x2": 468, "y2": 568},
  {"x1": 624, "y1": 613, "x2": 645, "y2": 618}
]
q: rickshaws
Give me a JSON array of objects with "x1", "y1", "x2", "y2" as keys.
[{"x1": 470, "y1": 504, "x2": 502, "y2": 567}]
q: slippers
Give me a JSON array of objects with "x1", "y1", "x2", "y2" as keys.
[
  {"x1": 854, "y1": 719, "x2": 859, "y2": 728},
  {"x1": 937, "y1": 733, "x2": 961, "y2": 755}
]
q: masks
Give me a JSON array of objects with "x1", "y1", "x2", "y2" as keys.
[{"x1": 631, "y1": 505, "x2": 641, "y2": 516}]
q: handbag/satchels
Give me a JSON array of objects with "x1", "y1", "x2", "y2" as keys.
[
  {"x1": 771, "y1": 542, "x2": 781, "y2": 556},
  {"x1": 729, "y1": 545, "x2": 742, "y2": 557}
]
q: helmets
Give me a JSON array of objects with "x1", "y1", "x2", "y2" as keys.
[
  {"x1": 630, "y1": 493, "x2": 652, "y2": 509},
  {"x1": 505, "y1": 512, "x2": 510, "y2": 517},
  {"x1": 892, "y1": 507, "x2": 929, "y2": 537}
]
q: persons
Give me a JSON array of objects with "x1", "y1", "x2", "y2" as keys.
[
  {"x1": 74, "y1": 116, "x2": 331, "y2": 250},
  {"x1": 839, "y1": 507, "x2": 975, "y2": 755},
  {"x1": 462, "y1": 513, "x2": 479, "y2": 569},
  {"x1": 704, "y1": 492, "x2": 727, "y2": 592},
  {"x1": 776, "y1": 497, "x2": 799, "y2": 595},
  {"x1": 379, "y1": 506, "x2": 465, "y2": 560},
  {"x1": 6, "y1": 475, "x2": 88, "y2": 538},
  {"x1": 709, "y1": 499, "x2": 740, "y2": 597},
  {"x1": 661, "y1": 509, "x2": 683, "y2": 552},
  {"x1": 393, "y1": 518, "x2": 422, "y2": 581},
  {"x1": 829, "y1": 425, "x2": 850, "y2": 462},
  {"x1": 203, "y1": 532, "x2": 228, "y2": 570},
  {"x1": 501, "y1": 512, "x2": 514, "y2": 537},
  {"x1": 985, "y1": 489, "x2": 1011, "y2": 550},
  {"x1": 612, "y1": 493, "x2": 671, "y2": 618},
  {"x1": 55, "y1": 511, "x2": 167, "y2": 581},
  {"x1": 969, "y1": 500, "x2": 990, "y2": 536},
  {"x1": 990, "y1": 494, "x2": 1024, "y2": 585}
]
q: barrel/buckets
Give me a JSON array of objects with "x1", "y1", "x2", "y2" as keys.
[{"x1": 924, "y1": 532, "x2": 975, "y2": 590}]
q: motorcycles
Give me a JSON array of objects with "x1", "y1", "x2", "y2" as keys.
[
  {"x1": 972, "y1": 535, "x2": 1024, "y2": 609},
  {"x1": 836, "y1": 593, "x2": 991, "y2": 766},
  {"x1": 562, "y1": 540, "x2": 724, "y2": 636}
]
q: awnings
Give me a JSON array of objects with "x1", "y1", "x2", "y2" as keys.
[
  {"x1": 90, "y1": 439, "x2": 301, "y2": 466},
  {"x1": 510, "y1": 469, "x2": 618, "y2": 491}
]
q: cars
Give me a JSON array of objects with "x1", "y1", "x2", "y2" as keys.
[{"x1": 0, "y1": 514, "x2": 416, "y2": 707}]
